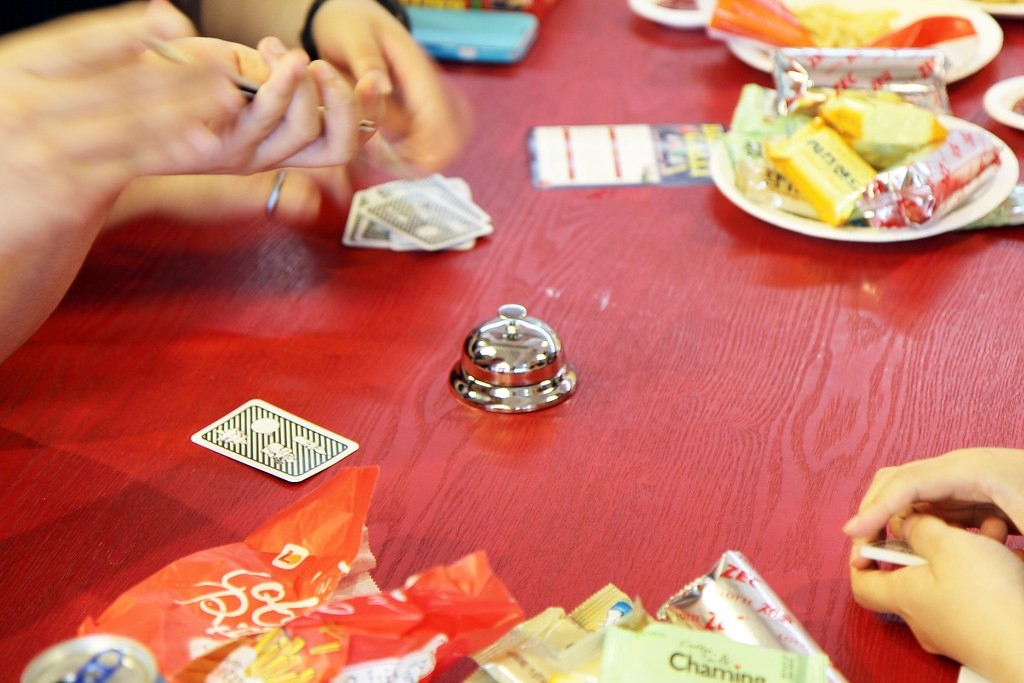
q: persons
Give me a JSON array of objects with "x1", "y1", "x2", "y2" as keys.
[
  {"x1": 0, "y1": 0, "x2": 476, "y2": 356},
  {"x1": 841, "y1": 446, "x2": 1024, "y2": 683}
]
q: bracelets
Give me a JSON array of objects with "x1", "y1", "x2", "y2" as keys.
[{"x1": 301, "y1": 0, "x2": 325, "y2": 61}]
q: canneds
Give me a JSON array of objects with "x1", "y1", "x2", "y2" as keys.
[{"x1": 19, "y1": 635, "x2": 167, "y2": 683}]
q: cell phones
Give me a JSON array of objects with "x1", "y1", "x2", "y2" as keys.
[{"x1": 405, "y1": 7, "x2": 539, "y2": 63}]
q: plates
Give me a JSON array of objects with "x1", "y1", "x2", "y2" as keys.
[
  {"x1": 981, "y1": 75, "x2": 1024, "y2": 130},
  {"x1": 711, "y1": 115, "x2": 1020, "y2": 242},
  {"x1": 728, "y1": 0, "x2": 1003, "y2": 85}
]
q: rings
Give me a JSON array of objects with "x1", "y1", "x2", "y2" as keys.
[{"x1": 266, "y1": 172, "x2": 285, "y2": 214}]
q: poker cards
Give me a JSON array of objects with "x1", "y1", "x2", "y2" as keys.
[
  {"x1": 858, "y1": 535, "x2": 929, "y2": 571},
  {"x1": 137, "y1": 28, "x2": 377, "y2": 136},
  {"x1": 189, "y1": 397, "x2": 360, "y2": 484},
  {"x1": 341, "y1": 164, "x2": 496, "y2": 254}
]
